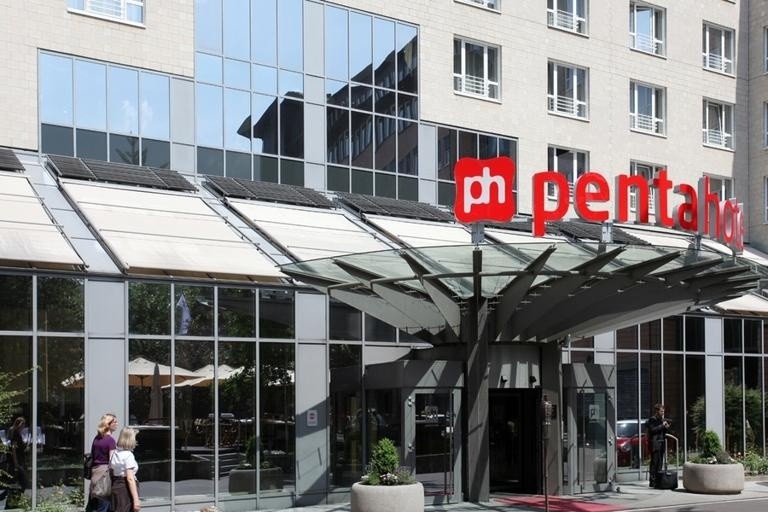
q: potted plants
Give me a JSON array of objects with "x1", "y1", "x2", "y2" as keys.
[
  {"x1": 226, "y1": 434, "x2": 285, "y2": 494},
  {"x1": 681, "y1": 427, "x2": 744, "y2": 495},
  {"x1": 350, "y1": 435, "x2": 425, "y2": 512}
]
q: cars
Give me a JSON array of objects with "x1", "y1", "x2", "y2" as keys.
[
  {"x1": 615, "y1": 418, "x2": 675, "y2": 469},
  {"x1": 416, "y1": 413, "x2": 454, "y2": 436}
]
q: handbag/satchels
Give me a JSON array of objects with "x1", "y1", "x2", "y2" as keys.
[
  {"x1": 92, "y1": 469, "x2": 112, "y2": 497},
  {"x1": 83, "y1": 453, "x2": 93, "y2": 480}
]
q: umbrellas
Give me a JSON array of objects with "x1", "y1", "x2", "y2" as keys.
[{"x1": 61, "y1": 356, "x2": 295, "y2": 453}]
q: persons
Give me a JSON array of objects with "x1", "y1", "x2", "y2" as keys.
[
  {"x1": 5, "y1": 417, "x2": 29, "y2": 509},
  {"x1": 85, "y1": 413, "x2": 119, "y2": 512},
  {"x1": 647, "y1": 403, "x2": 672, "y2": 488},
  {"x1": 109, "y1": 426, "x2": 144, "y2": 512}
]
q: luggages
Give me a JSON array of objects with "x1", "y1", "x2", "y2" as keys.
[{"x1": 655, "y1": 438, "x2": 678, "y2": 489}]
